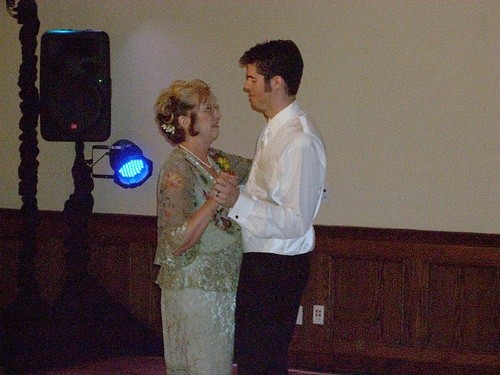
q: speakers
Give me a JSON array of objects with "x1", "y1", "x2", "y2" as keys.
[{"x1": 40, "y1": 29, "x2": 111, "y2": 143}]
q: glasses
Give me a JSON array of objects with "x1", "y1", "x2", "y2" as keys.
[{"x1": 192, "y1": 104, "x2": 222, "y2": 116}]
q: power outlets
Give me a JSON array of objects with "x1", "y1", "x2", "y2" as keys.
[
  {"x1": 312, "y1": 305, "x2": 324, "y2": 325},
  {"x1": 296, "y1": 306, "x2": 303, "y2": 325}
]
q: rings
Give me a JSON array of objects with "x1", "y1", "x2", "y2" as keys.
[{"x1": 216, "y1": 191, "x2": 220, "y2": 196}]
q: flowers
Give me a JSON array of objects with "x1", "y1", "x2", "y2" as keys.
[
  {"x1": 216, "y1": 156, "x2": 230, "y2": 172},
  {"x1": 161, "y1": 124, "x2": 176, "y2": 134}
]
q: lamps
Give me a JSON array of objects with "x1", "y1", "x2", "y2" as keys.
[{"x1": 109, "y1": 140, "x2": 154, "y2": 188}]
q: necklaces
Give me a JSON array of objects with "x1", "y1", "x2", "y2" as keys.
[{"x1": 178, "y1": 144, "x2": 213, "y2": 168}]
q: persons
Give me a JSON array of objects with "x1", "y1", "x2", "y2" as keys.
[
  {"x1": 210, "y1": 38, "x2": 326, "y2": 375},
  {"x1": 152, "y1": 79, "x2": 254, "y2": 375}
]
